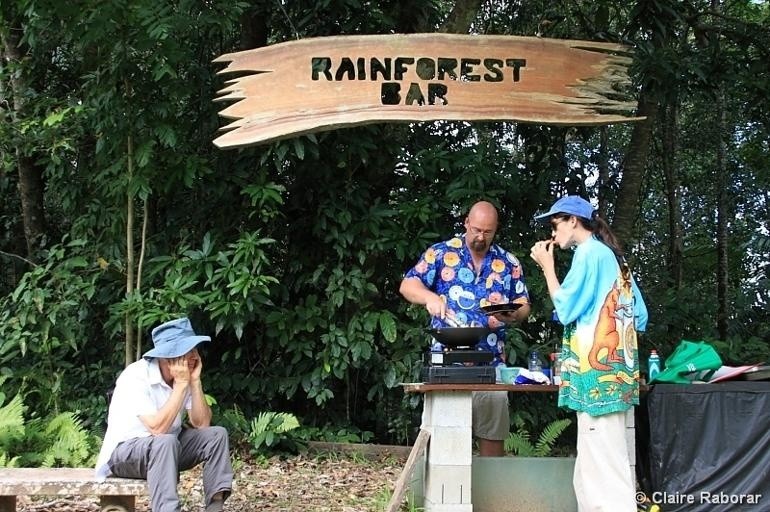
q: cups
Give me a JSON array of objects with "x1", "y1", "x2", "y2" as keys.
[{"x1": 501, "y1": 367, "x2": 520, "y2": 385}]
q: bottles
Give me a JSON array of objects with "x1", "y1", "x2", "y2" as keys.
[
  {"x1": 529, "y1": 352, "x2": 542, "y2": 373},
  {"x1": 550, "y1": 353, "x2": 562, "y2": 384},
  {"x1": 648, "y1": 350, "x2": 660, "y2": 382}
]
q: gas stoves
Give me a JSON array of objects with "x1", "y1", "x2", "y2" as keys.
[{"x1": 419, "y1": 344, "x2": 494, "y2": 367}]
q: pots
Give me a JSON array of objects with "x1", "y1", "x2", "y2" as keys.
[{"x1": 420, "y1": 326, "x2": 509, "y2": 348}]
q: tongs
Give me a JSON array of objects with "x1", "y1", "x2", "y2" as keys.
[{"x1": 443, "y1": 312, "x2": 470, "y2": 328}]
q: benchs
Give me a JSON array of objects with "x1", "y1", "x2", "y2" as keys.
[{"x1": 0, "y1": 468, "x2": 151, "y2": 512}]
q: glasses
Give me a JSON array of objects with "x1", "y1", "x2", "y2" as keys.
[
  {"x1": 468, "y1": 218, "x2": 495, "y2": 239},
  {"x1": 551, "y1": 221, "x2": 562, "y2": 231}
]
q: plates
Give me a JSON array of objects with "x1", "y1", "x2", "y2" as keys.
[{"x1": 478, "y1": 303, "x2": 524, "y2": 316}]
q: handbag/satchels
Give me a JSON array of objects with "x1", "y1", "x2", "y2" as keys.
[{"x1": 647, "y1": 339, "x2": 724, "y2": 386}]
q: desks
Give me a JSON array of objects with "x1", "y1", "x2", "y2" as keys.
[{"x1": 403, "y1": 383, "x2": 650, "y2": 512}]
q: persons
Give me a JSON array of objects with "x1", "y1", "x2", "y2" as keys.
[
  {"x1": 529, "y1": 195, "x2": 649, "y2": 511},
  {"x1": 94, "y1": 315, "x2": 235, "y2": 511},
  {"x1": 398, "y1": 200, "x2": 531, "y2": 457}
]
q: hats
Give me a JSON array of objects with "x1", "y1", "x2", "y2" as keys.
[
  {"x1": 142, "y1": 317, "x2": 213, "y2": 359},
  {"x1": 533, "y1": 194, "x2": 598, "y2": 223}
]
